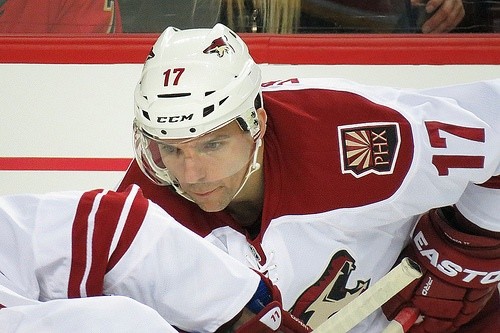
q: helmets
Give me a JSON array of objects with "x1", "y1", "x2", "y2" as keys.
[{"x1": 132, "y1": 21, "x2": 262, "y2": 187}]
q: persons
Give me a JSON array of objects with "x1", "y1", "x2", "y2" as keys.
[
  {"x1": 112, "y1": 21, "x2": 500, "y2": 333},
  {"x1": 0, "y1": 183, "x2": 312, "y2": 333},
  {"x1": 0, "y1": 0, "x2": 500, "y2": 36}
]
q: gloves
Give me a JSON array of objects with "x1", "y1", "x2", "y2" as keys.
[{"x1": 382, "y1": 208, "x2": 500, "y2": 333}]
